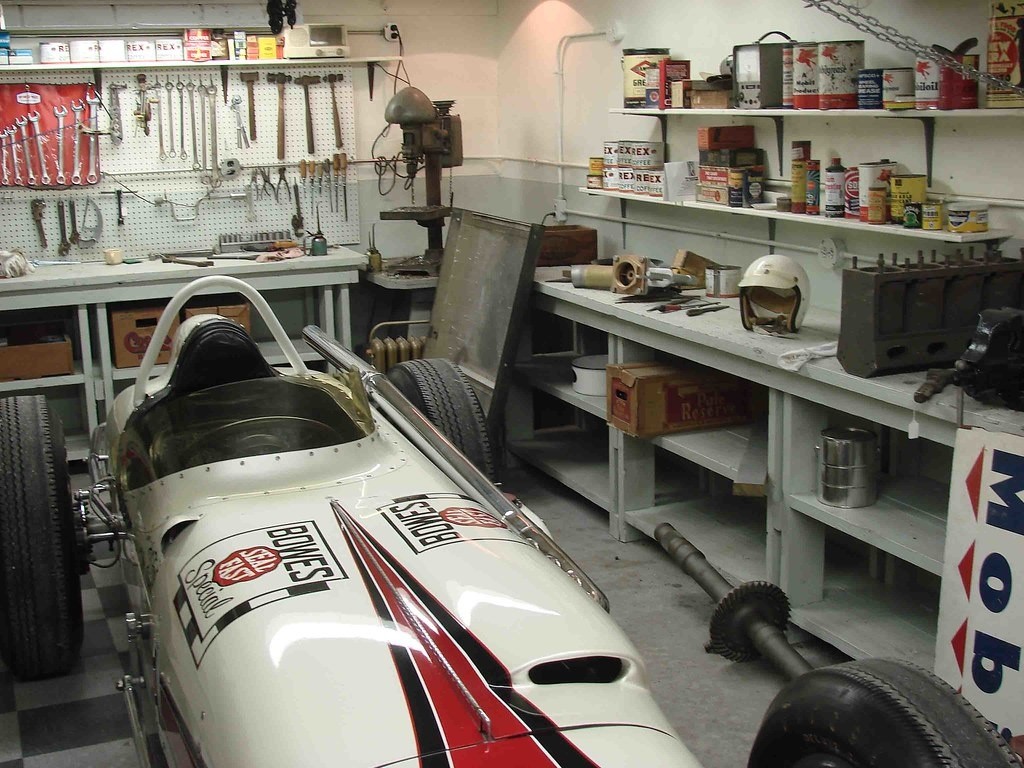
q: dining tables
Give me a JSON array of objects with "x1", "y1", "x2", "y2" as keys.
[{"x1": 699, "y1": 163, "x2": 764, "y2": 186}]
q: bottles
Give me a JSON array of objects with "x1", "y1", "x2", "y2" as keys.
[
  {"x1": 825, "y1": 157, "x2": 848, "y2": 218},
  {"x1": 369, "y1": 249, "x2": 382, "y2": 272},
  {"x1": 312, "y1": 234, "x2": 327, "y2": 255}
]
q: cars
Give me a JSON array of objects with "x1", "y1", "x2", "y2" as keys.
[{"x1": 0, "y1": 275, "x2": 1023, "y2": 768}]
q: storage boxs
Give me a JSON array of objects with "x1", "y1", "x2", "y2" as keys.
[
  {"x1": 603, "y1": 359, "x2": 764, "y2": 442},
  {"x1": 111, "y1": 306, "x2": 182, "y2": 371},
  {"x1": 97, "y1": 40, "x2": 127, "y2": 61},
  {"x1": 68, "y1": 40, "x2": 98, "y2": 62},
  {"x1": 183, "y1": 28, "x2": 210, "y2": 62},
  {"x1": 658, "y1": 58, "x2": 692, "y2": 111},
  {"x1": 696, "y1": 122, "x2": 756, "y2": 151},
  {"x1": 644, "y1": 67, "x2": 660, "y2": 109},
  {"x1": 124, "y1": 41, "x2": 156, "y2": 62},
  {"x1": 226, "y1": 30, "x2": 287, "y2": 60},
  {"x1": 698, "y1": 148, "x2": 765, "y2": 167},
  {"x1": 38, "y1": 41, "x2": 70, "y2": 63},
  {"x1": 184, "y1": 302, "x2": 253, "y2": 341},
  {"x1": 673, "y1": 248, "x2": 718, "y2": 277},
  {"x1": 536, "y1": 224, "x2": 599, "y2": 270},
  {"x1": 688, "y1": 90, "x2": 730, "y2": 109},
  {"x1": 668, "y1": 78, "x2": 691, "y2": 109},
  {"x1": 156, "y1": 38, "x2": 184, "y2": 62},
  {"x1": 695, "y1": 184, "x2": 730, "y2": 204},
  {"x1": 0, "y1": 332, "x2": 74, "y2": 384}
]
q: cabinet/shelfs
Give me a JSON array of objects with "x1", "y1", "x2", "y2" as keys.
[
  {"x1": 574, "y1": 101, "x2": 1024, "y2": 264},
  {"x1": 499, "y1": 253, "x2": 1024, "y2": 726}
]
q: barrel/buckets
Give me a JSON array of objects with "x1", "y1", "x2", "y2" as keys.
[
  {"x1": 814, "y1": 426, "x2": 882, "y2": 509},
  {"x1": 620, "y1": 47, "x2": 670, "y2": 109},
  {"x1": 781, "y1": 0, "x2": 1024, "y2": 108}
]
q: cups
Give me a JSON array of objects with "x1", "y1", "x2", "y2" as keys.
[
  {"x1": 705, "y1": 264, "x2": 741, "y2": 299},
  {"x1": 39, "y1": 38, "x2": 184, "y2": 64},
  {"x1": 777, "y1": 197, "x2": 791, "y2": 213},
  {"x1": 104, "y1": 248, "x2": 122, "y2": 264}
]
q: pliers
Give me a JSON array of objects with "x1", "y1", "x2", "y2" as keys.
[
  {"x1": 259, "y1": 168, "x2": 275, "y2": 197},
  {"x1": 276, "y1": 168, "x2": 291, "y2": 203}
]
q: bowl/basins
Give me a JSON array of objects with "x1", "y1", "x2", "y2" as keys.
[{"x1": 571, "y1": 353, "x2": 611, "y2": 397}]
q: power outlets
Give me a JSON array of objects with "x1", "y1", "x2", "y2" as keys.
[{"x1": 551, "y1": 198, "x2": 568, "y2": 223}]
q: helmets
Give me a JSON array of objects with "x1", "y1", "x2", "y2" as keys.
[{"x1": 737, "y1": 254, "x2": 810, "y2": 332}]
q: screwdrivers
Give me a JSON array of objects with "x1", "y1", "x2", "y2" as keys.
[
  {"x1": 333, "y1": 154, "x2": 338, "y2": 211},
  {"x1": 658, "y1": 305, "x2": 702, "y2": 313},
  {"x1": 301, "y1": 159, "x2": 306, "y2": 188},
  {"x1": 339, "y1": 153, "x2": 348, "y2": 221},
  {"x1": 326, "y1": 159, "x2": 333, "y2": 212},
  {"x1": 318, "y1": 162, "x2": 323, "y2": 193},
  {"x1": 309, "y1": 161, "x2": 315, "y2": 187}
]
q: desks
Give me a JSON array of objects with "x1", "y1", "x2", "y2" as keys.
[
  {"x1": 6, "y1": 238, "x2": 369, "y2": 467},
  {"x1": 357, "y1": 257, "x2": 439, "y2": 338}
]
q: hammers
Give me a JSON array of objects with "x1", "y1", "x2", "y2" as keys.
[
  {"x1": 266, "y1": 72, "x2": 292, "y2": 159},
  {"x1": 324, "y1": 73, "x2": 343, "y2": 148},
  {"x1": 240, "y1": 72, "x2": 260, "y2": 140},
  {"x1": 295, "y1": 76, "x2": 320, "y2": 154}
]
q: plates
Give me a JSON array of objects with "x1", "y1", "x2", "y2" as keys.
[{"x1": 750, "y1": 203, "x2": 776, "y2": 210}]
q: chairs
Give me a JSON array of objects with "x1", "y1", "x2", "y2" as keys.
[{"x1": 113, "y1": 312, "x2": 364, "y2": 489}]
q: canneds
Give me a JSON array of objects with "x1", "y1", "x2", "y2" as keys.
[
  {"x1": 586, "y1": 157, "x2": 603, "y2": 189},
  {"x1": 904, "y1": 202, "x2": 944, "y2": 230},
  {"x1": 868, "y1": 186, "x2": 887, "y2": 224}
]
